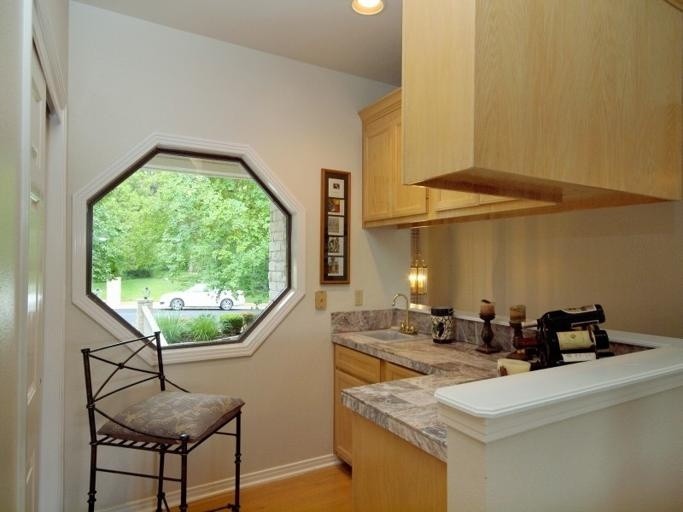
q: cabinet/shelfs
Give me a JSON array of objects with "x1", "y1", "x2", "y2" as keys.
[
  {"x1": 331, "y1": 346, "x2": 381, "y2": 467},
  {"x1": 359, "y1": 105, "x2": 428, "y2": 224}
]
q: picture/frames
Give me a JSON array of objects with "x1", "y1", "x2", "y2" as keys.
[{"x1": 318, "y1": 166, "x2": 352, "y2": 286}]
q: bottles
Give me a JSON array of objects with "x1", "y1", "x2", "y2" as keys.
[
  {"x1": 511, "y1": 304, "x2": 615, "y2": 371},
  {"x1": 430, "y1": 306, "x2": 455, "y2": 344}
]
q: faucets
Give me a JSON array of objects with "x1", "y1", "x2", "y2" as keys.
[{"x1": 391, "y1": 292, "x2": 419, "y2": 336}]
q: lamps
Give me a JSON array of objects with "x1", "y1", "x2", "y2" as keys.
[{"x1": 407, "y1": 245, "x2": 429, "y2": 296}]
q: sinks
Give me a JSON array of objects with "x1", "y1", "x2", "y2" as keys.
[{"x1": 363, "y1": 329, "x2": 432, "y2": 344}]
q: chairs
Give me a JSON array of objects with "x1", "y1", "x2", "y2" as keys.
[{"x1": 78, "y1": 330, "x2": 245, "y2": 510}]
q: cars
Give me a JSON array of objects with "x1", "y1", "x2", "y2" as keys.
[{"x1": 160, "y1": 283, "x2": 245, "y2": 310}]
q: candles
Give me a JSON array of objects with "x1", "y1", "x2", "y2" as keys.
[
  {"x1": 507, "y1": 304, "x2": 526, "y2": 322},
  {"x1": 479, "y1": 301, "x2": 495, "y2": 314}
]
